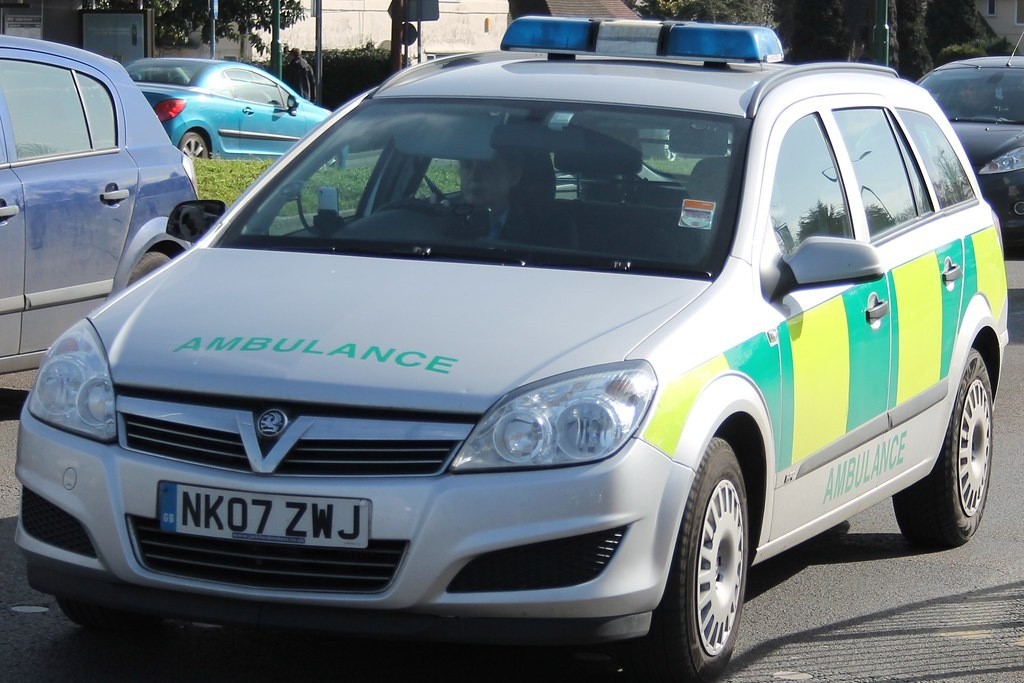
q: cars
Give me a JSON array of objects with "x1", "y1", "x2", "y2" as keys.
[
  {"x1": 15, "y1": 19, "x2": 1011, "y2": 683},
  {"x1": 107, "y1": 56, "x2": 350, "y2": 172},
  {"x1": 0, "y1": 37, "x2": 201, "y2": 374},
  {"x1": 852, "y1": 56, "x2": 1024, "y2": 226}
]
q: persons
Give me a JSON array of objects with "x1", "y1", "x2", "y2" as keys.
[
  {"x1": 436, "y1": 137, "x2": 536, "y2": 254},
  {"x1": 283, "y1": 47, "x2": 317, "y2": 106}
]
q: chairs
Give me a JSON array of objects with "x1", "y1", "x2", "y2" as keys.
[
  {"x1": 508, "y1": 143, "x2": 575, "y2": 244},
  {"x1": 654, "y1": 157, "x2": 733, "y2": 261}
]
